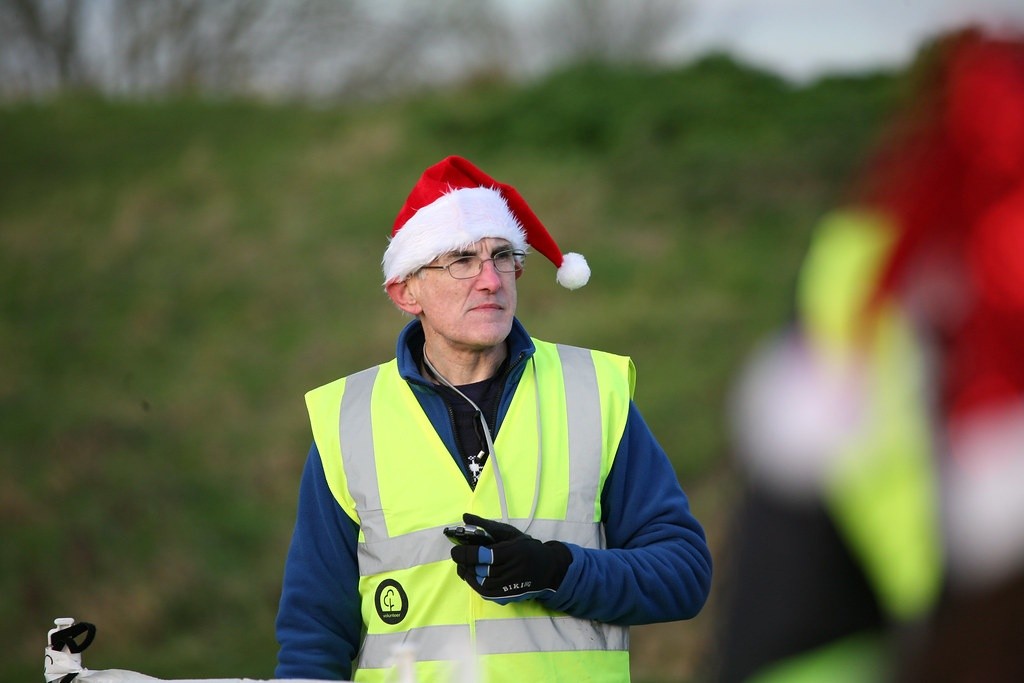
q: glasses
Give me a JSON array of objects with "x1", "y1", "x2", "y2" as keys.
[{"x1": 419, "y1": 249, "x2": 526, "y2": 281}]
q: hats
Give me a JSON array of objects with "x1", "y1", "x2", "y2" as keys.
[{"x1": 379, "y1": 156, "x2": 591, "y2": 289}]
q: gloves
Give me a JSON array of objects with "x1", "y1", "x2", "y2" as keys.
[{"x1": 451, "y1": 513, "x2": 568, "y2": 604}]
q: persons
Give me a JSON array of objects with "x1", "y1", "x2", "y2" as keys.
[
  {"x1": 749, "y1": 23, "x2": 1024, "y2": 683},
  {"x1": 276, "y1": 154, "x2": 713, "y2": 683}
]
